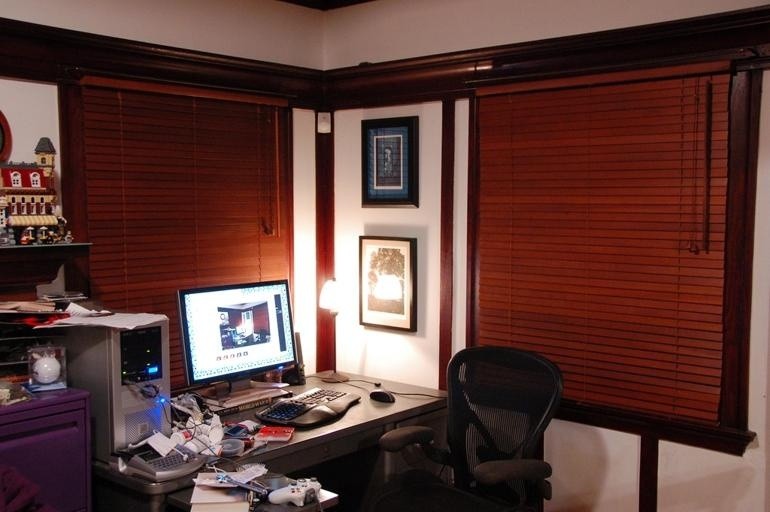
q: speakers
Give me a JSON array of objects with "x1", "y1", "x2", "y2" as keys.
[
  {"x1": 281, "y1": 332, "x2": 306, "y2": 385},
  {"x1": 317, "y1": 112, "x2": 332, "y2": 134}
]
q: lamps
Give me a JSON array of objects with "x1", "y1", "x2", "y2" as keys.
[{"x1": 318, "y1": 276, "x2": 350, "y2": 383}]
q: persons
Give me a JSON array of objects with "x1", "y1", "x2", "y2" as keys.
[{"x1": 377, "y1": 146, "x2": 400, "y2": 186}]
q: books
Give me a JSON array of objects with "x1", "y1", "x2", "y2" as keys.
[
  {"x1": 252, "y1": 424, "x2": 296, "y2": 444},
  {"x1": 190, "y1": 471, "x2": 252, "y2": 511}
]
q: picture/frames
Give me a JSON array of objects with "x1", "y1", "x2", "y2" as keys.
[
  {"x1": 359, "y1": 235, "x2": 417, "y2": 331},
  {"x1": 361, "y1": 115, "x2": 419, "y2": 209}
]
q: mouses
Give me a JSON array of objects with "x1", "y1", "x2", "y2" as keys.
[{"x1": 369, "y1": 389, "x2": 395, "y2": 403}]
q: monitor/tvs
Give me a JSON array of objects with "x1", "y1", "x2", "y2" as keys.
[{"x1": 177, "y1": 279, "x2": 298, "y2": 409}]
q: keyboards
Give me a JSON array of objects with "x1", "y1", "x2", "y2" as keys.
[{"x1": 256, "y1": 387, "x2": 361, "y2": 427}]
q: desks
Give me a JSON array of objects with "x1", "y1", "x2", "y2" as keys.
[{"x1": 90, "y1": 370, "x2": 452, "y2": 512}]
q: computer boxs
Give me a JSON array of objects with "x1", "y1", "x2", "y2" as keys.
[{"x1": 67, "y1": 318, "x2": 172, "y2": 464}]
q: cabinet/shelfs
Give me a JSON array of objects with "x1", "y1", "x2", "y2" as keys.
[
  {"x1": 0, "y1": 241, "x2": 94, "y2": 387},
  {"x1": 0, "y1": 387, "x2": 95, "y2": 512}
]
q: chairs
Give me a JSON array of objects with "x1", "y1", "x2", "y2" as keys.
[{"x1": 367, "y1": 346, "x2": 564, "y2": 512}]
